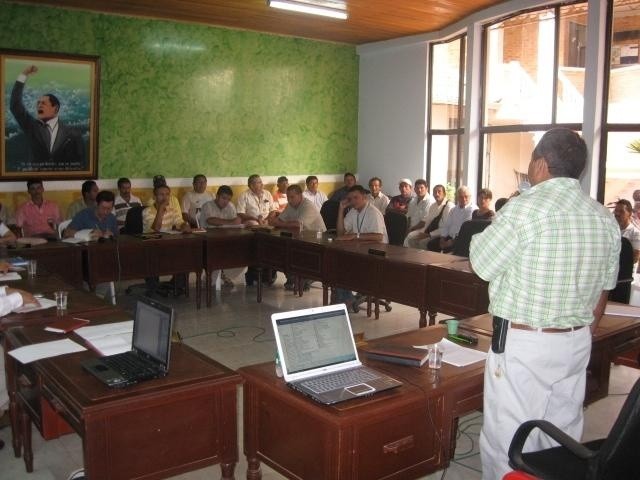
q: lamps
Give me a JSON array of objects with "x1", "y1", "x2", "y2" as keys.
[{"x1": 267, "y1": 1, "x2": 349, "y2": 22}]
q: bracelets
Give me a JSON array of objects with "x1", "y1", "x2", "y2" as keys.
[{"x1": 356, "y1": 233, "x2": 361, "y2": 239}]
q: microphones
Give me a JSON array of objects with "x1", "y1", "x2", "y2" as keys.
[{"x1": 507, "y1": 182, "x2": 530, "y2": 200}]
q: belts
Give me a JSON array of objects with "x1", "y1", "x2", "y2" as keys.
[{"x1": 510, "y1": 324, "x2": 581, "y2": 333}]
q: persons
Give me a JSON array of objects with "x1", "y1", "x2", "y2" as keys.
[
  {"x1": 198, "y1": 185, "x2": 242, "y2": 287},
  {"x1": 9, "y1": 64, "x2": 85, "y2": 171},
  {"x1": 181, "y1": 174, "x2": 218, "y2": 223},
  {"x1": 111, "y1": 177, "x2": 142, "y2": 234},
  {"x1": 0, "y1": 202, "x2": 16, "y2": 248},
  {"x1": 67, "y1": 180, "x2": 99, "y2": 220},
  {"x1": 146, "y1": 175, "x2": 180, "y2": 209},
  {"x1": 277, "y1": 169, "x2": 507, "y2": 258},
  {"x1": 0, "y1": 262, "x2": 16, "y2": 274},
  {"x1": 613, "y1": 199, "x2": 639, "y2": 268},
  {"x1": 62, "y1": 191, "x2": 132, "y2": 297},
  {"x1": 279, "y1": 185, "x2": 327, "y2": 292},
  {"x1": 334, "y1": 185, "x2": 390, "y2": 314},
  {"x1": 141, "y1": 186, "x2": 192, "y2": 296},
  {"x1": 0, "y1": 285, "x2": 40, "y2": 318},
  {"x1": 14, "y1": 178, "x2": 62, "y2": 236},
  {"x1": 467, "y1": 127, "x2": 622, "y2": 480},
  {"x1": 239, "y1": 175, "x2": 279, "y2": 285}
]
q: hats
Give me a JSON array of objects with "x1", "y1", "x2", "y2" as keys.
[{"x1": 399, "y1": 179, "x2": 412, "y2": 187}]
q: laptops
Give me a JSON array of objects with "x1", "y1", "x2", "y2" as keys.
[
  {"x1": 79, "y1": 293, "x2": 173, "y2": 388},
  {"x1": 271, "y1": 303, "x2": 404, "y2": 407}
]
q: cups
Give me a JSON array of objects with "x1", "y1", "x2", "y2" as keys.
[
  {"x1": 446, "y1": 319, "x2": 459, "y2": 335},
  {"x1": 428, "y1": 343, "x2": 444, "y2": 370},
  {"x1": 53, "y1": 290, "x2": 69, "y2": 310},
  {"x1": 27, "y1": 259, "x2": 37, "y2": 274},
  {"x1": 316, "y1": 228, "x2": 323, "y2": 239}
]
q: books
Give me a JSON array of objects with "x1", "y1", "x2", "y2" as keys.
[
  {"x1": 138, "y1": 232, "x2": 162, "y2": 242},
  {"x1": 359, "y1": 349, "x2": 431, "y2": 367},
  {"x1": 16, "y1": 236, "x2": 47, "y2": 247},
  {"x1": 0, "y1": 256, "x2": 29, "y2": 266},
  {"x1": 73, "y1": 319, "x2": 135, "y2": 357},
  {"x1": 191, "y1": 226, "x2": 206, "y2": 234},
  {"x1": 359, "y1": 339, "x2": 429, "y2": 362},
  {"x1": 601, "y1": 304, "x2": 640, "y2": 319},
  {"x1": 44, "y1": 318, "x2": 90, "y2": 335}
]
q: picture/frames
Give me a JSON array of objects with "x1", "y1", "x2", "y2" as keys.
[{"x1": 0, "y1": 49, "x2": 99, "y2": 180}]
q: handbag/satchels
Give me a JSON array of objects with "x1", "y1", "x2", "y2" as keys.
[{"x1": 424, "y1": 215, "x2": 441, "y2": 233}]
